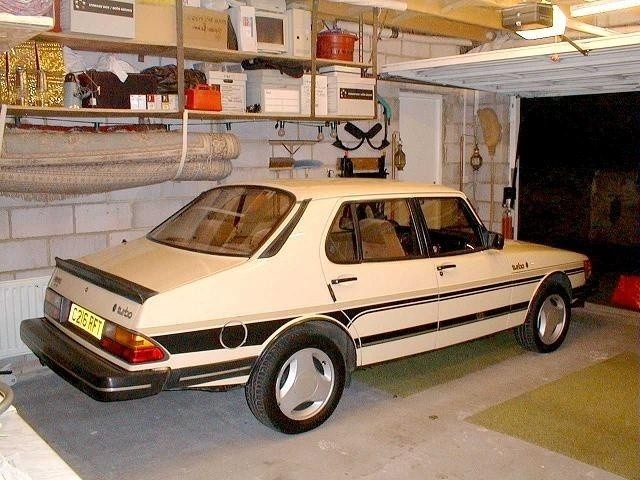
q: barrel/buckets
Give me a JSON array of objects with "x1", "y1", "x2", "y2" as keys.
[{"x1": 317, "y1": 28, "x2": 361, "y2": 63}]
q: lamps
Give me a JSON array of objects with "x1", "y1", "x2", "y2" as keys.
[
  {"x1": 459, "y1": 134, "x2": 482, "y2": 192},
  {"x1": 570, "y1": 0, "x2": 640, "y2": 18},
  {"x1": 501, "y1": 3, "x2": 588, "y2": 62},
  {"x1": 392, "y1": 131, "x2": 406, "y2": 180}
]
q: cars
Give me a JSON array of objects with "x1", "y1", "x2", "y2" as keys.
[{"x1": 20, "y1": 178, "x2": 593, "y2": 435}]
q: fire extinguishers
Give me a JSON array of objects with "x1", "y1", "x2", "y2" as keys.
[{"x1": 502, "y1": 203, "x2": 513, "y2": 240}]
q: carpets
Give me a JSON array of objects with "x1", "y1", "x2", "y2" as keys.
[
  {"x1": 462, "y1": 351, "x2": 640, "y2": 480},
  {"x1": 351, "y1": 331, "x2": 526, "y2": 398}
]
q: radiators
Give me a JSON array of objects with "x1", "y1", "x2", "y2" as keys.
[{"x1": 0, "y1": 274, "x2": 52, "y2": 359}]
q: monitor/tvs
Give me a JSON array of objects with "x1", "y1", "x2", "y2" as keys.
[{"x1": 255, "y1": 10, "x2": 288, "y2": 54}]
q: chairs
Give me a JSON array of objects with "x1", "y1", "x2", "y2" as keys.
[{"x1": 195, "y1": 219, "x2": 405, "y2": 262}]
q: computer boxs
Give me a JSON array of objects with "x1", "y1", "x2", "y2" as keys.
[{"x1": 286, "y1": 8, "x2": 311, "y2": 58}]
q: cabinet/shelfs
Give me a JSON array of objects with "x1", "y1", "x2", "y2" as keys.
[{"x1": 0, "y1": 13, "x2": 378, "y2": 121}]
